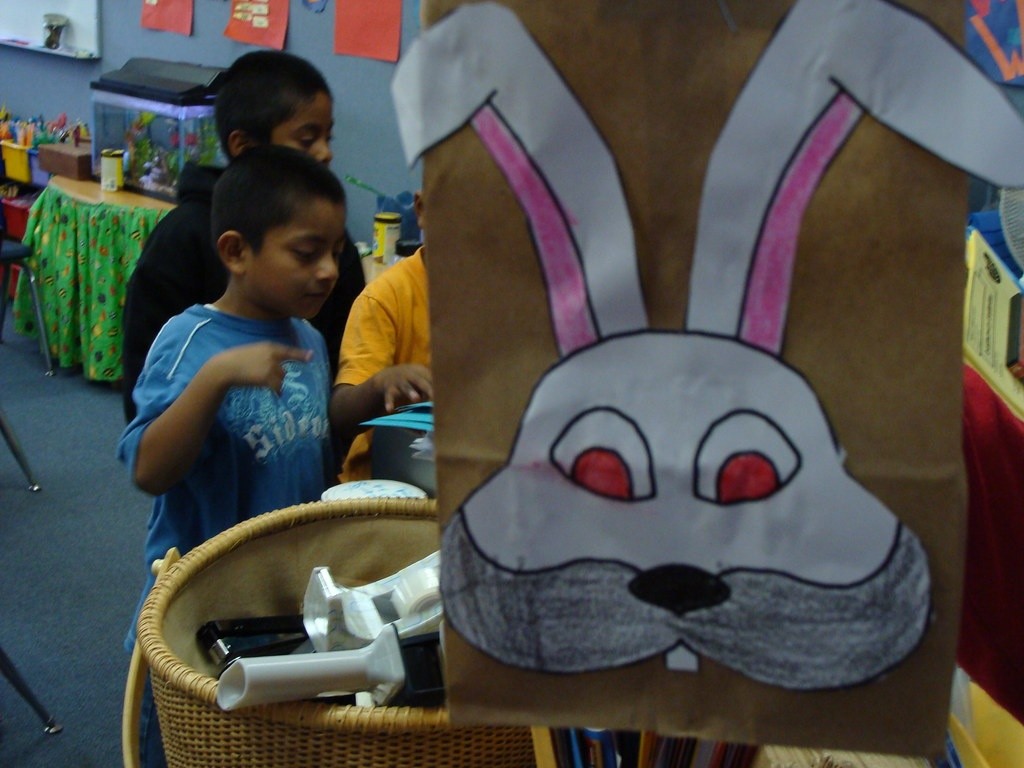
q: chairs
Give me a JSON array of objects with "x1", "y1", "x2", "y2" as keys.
[{"x1": 0, "y1": 202, "x2": 56, "y2": 377}]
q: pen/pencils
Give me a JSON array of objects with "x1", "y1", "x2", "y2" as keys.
[{"x1": 0, "y1": 102, "x2": 90, "y2": 146}]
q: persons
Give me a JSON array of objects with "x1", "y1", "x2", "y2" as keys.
[
  {"x1": 329, "y1": 184, "x2": 433, "y2": 485},
  {"x1": 121, "y1": 50, "x2": 367, "y2": 475},
  {"x1": 115, "y1": 145, "x2": 344, "y2": 768}
]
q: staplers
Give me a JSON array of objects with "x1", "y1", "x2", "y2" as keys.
[{"x1": 195, "y1": 613, "x2": 308, "y2": 678}]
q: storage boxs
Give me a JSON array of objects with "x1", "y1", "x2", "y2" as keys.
[
  {"x1": 90, "y1": 58, "x2": 229, "y2": 205},
  {"x1": 27, "y1": 148, "x2": 64, "y2": 184},
  {"x1": 0, "y1": 138, "x2": 34, "y2": 182},
  {"x1": 39, "y1": 141, "x2": 91, "y2": 180}
]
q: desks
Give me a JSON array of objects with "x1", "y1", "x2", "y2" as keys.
[{"x1": 11, "y1": 174, "x2": 178, "y2": 381}]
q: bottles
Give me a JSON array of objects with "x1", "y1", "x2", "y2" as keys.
[
  {"x1": 100, "y1": 149, "x2": 124, "y2": 194},
  {"x1": 372, "y1": 212, "x2": 402, "y2": 266}
]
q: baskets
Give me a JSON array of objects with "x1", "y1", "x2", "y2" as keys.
[{"x1": 124, "y1": 498, "x2": 537, "y2": 768}]
